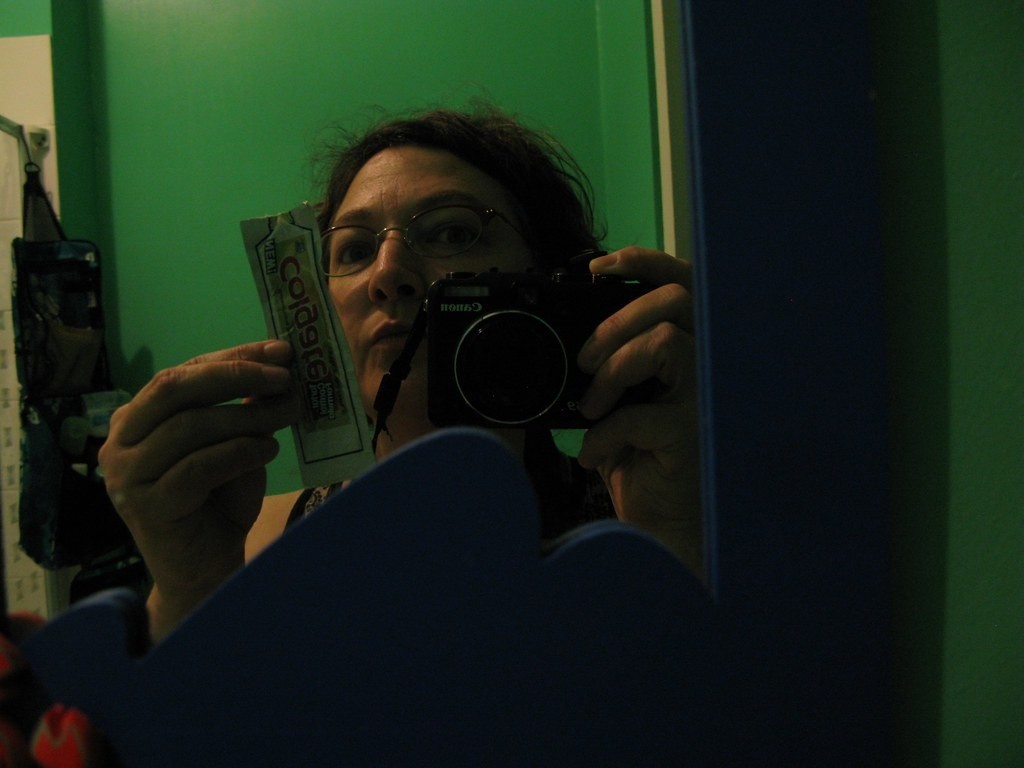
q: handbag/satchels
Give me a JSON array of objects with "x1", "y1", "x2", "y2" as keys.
[{"x1": 10, "y1": 134, "x2": 139, "y2": 573}]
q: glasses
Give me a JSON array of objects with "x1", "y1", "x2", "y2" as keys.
[{"x1": 320, "y1": 204, "x2": 529, "y2": 278}]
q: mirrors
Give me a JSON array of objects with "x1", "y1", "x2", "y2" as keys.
[{"x1": 0, "y1": 0, "x2": 889, "y2": 768}]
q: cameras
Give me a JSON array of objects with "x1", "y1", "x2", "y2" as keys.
[{"x1": 422, "y1": 273, "x2": 666, "y2": 433}]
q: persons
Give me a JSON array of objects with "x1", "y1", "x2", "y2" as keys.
[{"x1": 98, "y1": 106, "x2": 706, "y2": 649}]
